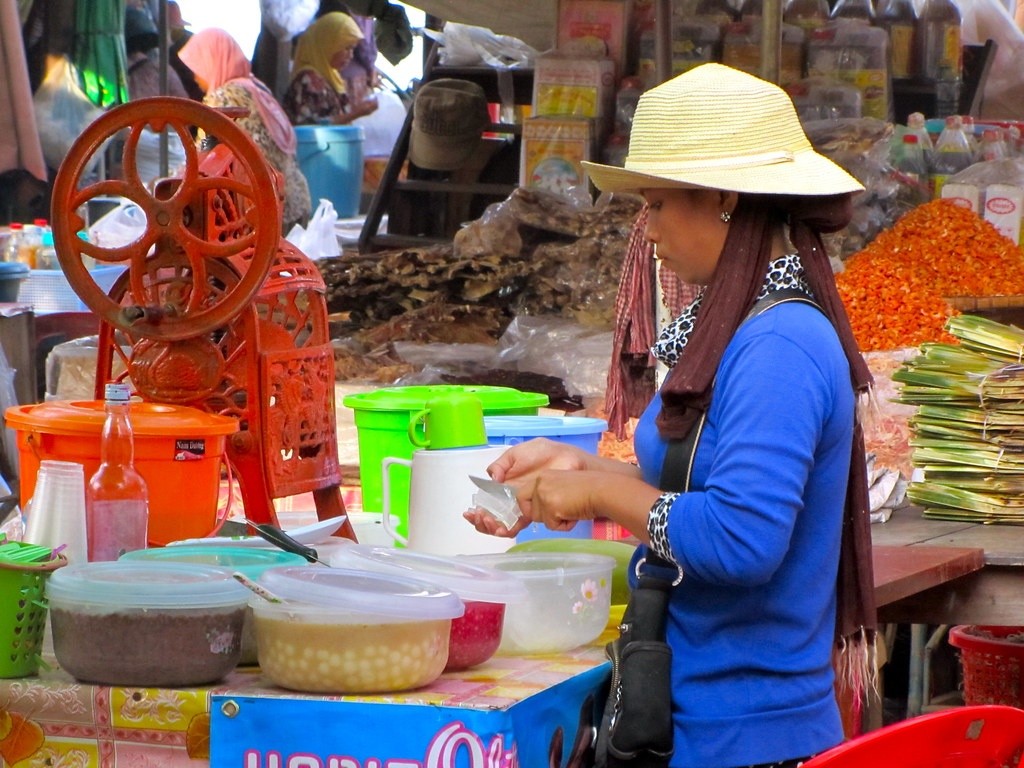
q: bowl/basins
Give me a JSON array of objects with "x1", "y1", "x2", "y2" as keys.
[{"x1": 42, "y1": 544, "x2": 616, "y2": 693}]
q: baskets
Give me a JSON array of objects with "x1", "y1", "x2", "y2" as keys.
[
  {"x1": 949, "y1": 624, "x2": 1023, "y2": 708},
  {"x1": 0, "y1": 533, "x2": 69, "y2": 678}
]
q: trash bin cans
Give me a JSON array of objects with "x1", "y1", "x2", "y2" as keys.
[{"x1": 948, "y1": 623, "x2": 1024, "y2": 710}]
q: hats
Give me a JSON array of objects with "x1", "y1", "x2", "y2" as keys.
[
  {"x1": 405, "y1": 77, "x2": 490, "y2": 170},
  {"x1": 166, "y1": 1, "x2": 193, "y2": 28},
  {"x1": 581, "y1": 62, "x2": 866, "y2": 197}
]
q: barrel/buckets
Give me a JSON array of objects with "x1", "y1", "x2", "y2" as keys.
[
  {"x1": 293, "y1": 125, "x2": 364, "y2": 220},
  {"x1": 5, "y1": 400, "x2": 239, "y2": 562},
  {"x1": 344, "y1": 384, "x2": 607, "y2": 542},
  {"x1": 798, "y1": 705, "x2": 1024, "y2": 768}
]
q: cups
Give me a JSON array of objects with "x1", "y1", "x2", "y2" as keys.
[
  {"x1": 24, "y1": 459, "x2": 85, "y2": 658},
  {"x1": 383, "y1": 445, "x2": 516, "y2": 559},
  {"x1": 409, "y1": 395, "x2": 488, "y2": 449}
]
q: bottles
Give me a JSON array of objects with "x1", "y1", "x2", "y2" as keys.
[
  {"x1": 4, "y1": 219, "x2": 63, "y2": 270},
  {"x1": 76, "y1": 232, "x2": 96, "y2": 268},
  {"x1": 695, "y1": 0, "x2": 962, "y2": 117},
  {"x1": 86, "y1": 383, "x2": 147, "y2": 562},
  {"x1": 891, "y1": 112, "x2": 1024, "y2": 201}
]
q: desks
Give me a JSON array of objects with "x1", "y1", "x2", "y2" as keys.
[{"x1": 0, "y1": 544, "x2": 988, "y2": 768}]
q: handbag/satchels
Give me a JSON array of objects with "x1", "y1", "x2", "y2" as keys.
[{"x1": 596, "y1": 554, "x2": 687, "y2": 768}]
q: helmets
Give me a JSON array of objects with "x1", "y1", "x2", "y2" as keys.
[{"x1": 124, "y1": 8, "x2": 162, "y2": 46}]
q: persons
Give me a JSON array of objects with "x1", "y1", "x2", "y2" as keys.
[
  {"x1": 463, "y1": 63, "x2": 866, "y2": 768},
  {"x1": 123, "y1": 0, "x2": 379, "y2": 266}
]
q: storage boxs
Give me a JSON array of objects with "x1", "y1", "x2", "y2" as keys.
[{"x1": 28, "y1": 264, "x2": 127, "y2": 316}]
